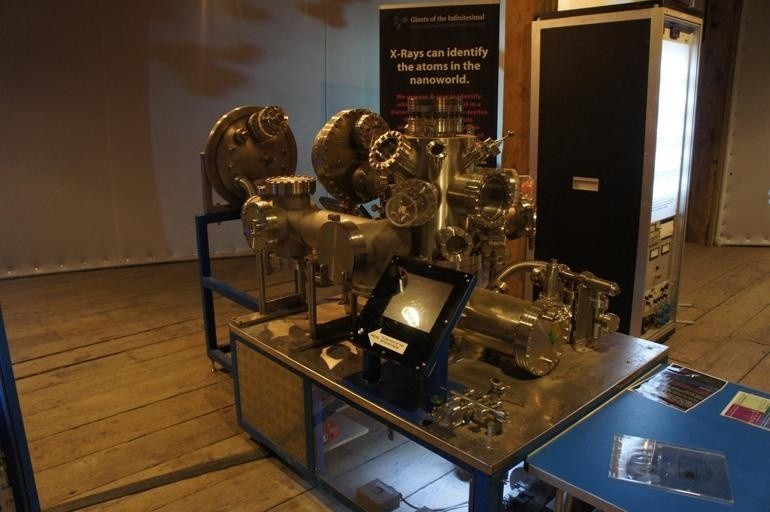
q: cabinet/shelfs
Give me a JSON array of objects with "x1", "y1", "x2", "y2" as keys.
[{"x1": 229, "y1": 308, "x2": 669, "y2": 512}]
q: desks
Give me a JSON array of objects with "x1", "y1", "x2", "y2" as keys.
[{"x1": 523, "y1": 364, "x2": 770, "y2": 512}]
